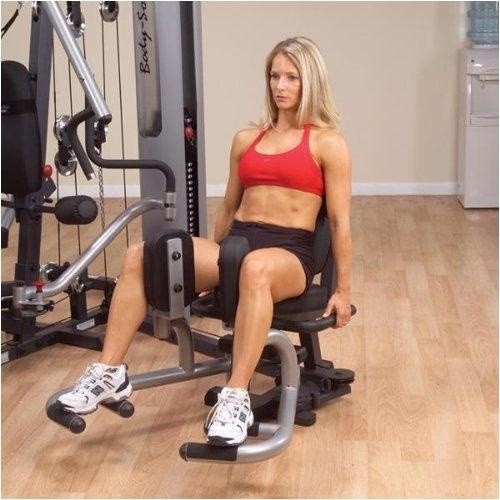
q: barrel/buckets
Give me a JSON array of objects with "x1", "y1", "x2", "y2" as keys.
[{"x1": 466, "y1": 1, "x2": 499, "y2": 47}]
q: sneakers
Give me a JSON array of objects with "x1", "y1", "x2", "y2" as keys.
[
  {"x1": 57, "y1": 363, "x2": 133, "y2": 414},
  {"x1": 207, "y1": 387, "x2": 254, "y2": 446}
]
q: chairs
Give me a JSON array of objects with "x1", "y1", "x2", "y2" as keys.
[{"x1": 46, "y1": 194, "x2": 357, "y2": 463}]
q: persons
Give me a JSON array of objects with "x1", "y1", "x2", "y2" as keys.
[{"x1": 57, "y1": 36, "x2": 353, "y2": 448}]
